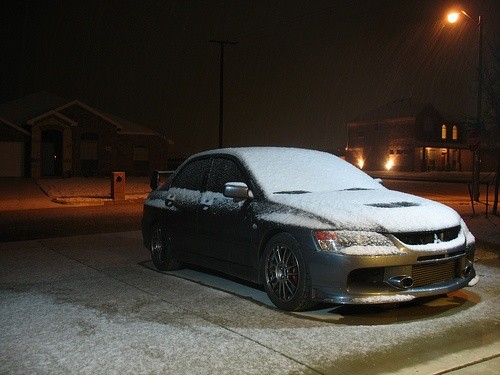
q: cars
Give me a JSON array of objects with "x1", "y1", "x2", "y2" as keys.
[{"x1": 140, "y1": 145, "x2": 480, "y2": 314}]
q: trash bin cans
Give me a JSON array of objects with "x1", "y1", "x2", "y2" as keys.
[{"x1": 110, "y1": 171, "x2": 126, "y2": 200}]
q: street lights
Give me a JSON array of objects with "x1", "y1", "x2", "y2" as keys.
[{"x1": 444, "y1": 6, "x2": 485, "y2": 201}]
